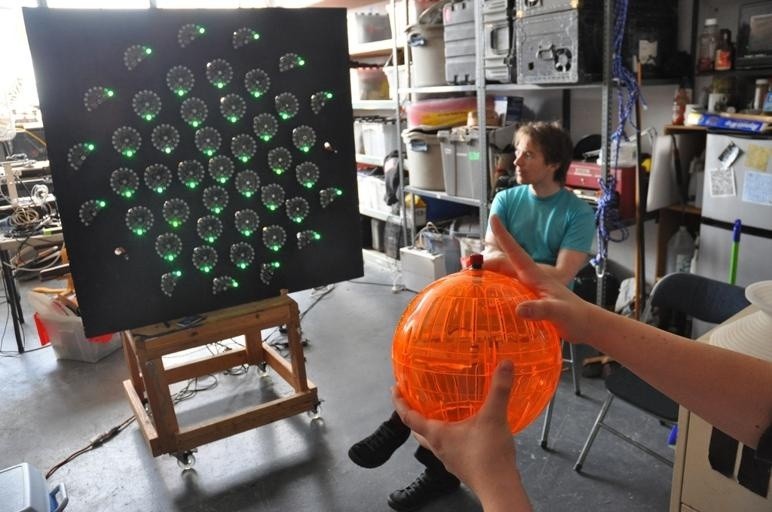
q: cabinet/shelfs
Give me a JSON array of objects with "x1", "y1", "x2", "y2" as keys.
[
  {"x1": 408, "y1": 2, "x2": 696, "y2": 310},
  {"x1": 667, "y1": 300, "x2": 772, "y2": 512},
  {"x1": 0, "y1": 118, "x2": 54, "y2": 189},
  {"x1": 666, "y1": 55, "x2": 771, "y2": 217},
  {"x1": 120, "y1": 294, "x2": 323, "y2": 469},
  {"x1": 346, "y1": 37, "x2": 406, "y2": 268}
]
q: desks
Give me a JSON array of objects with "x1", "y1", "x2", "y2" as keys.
[{"x1": 0, "y1": 217, "x2": 64, "y2": 354}]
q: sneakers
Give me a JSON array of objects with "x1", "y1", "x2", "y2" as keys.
[
  {"x1": 387, "y1": 468, "x2": 460, "y2": 512},
  {"x1": 348, "y1": 421, "x2": 405, "y2": 468}
]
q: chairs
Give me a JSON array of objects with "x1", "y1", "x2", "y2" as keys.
[
  {"x1": 574, "y1": 272, "x2": 750, "y2": 474},
  {"x1": 536, "y1": 273, "x2": 623, "y2": 449}
]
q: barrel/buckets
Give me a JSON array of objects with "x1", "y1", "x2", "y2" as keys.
[
  {"x1": 404, "y1": 23, "x2": 446, "y2": 88},
  {"x1": 400, "y1": 129, "x2": 446, "y2": 191}
]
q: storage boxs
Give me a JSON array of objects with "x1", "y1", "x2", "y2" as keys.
[
  {"x1": 436, "y1": 121, "x2": 519, "y2": 200},
  {"x1": 34, "y1": 292, "x2": 124, "y2": 364},
  {"x1": 0, "y1": 463, "x2": 69, "y2": 512}
]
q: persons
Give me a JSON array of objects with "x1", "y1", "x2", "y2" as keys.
[
  {"x1": 386, "y1": 211, "x2": 771, "y2": 511},
  {"x1": 345, "y1": 115, "x2": 598, "y2": 512}
]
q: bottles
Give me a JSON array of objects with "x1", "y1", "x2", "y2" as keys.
[
  {"x1": 754, "y1": 79, "x2": 771, "y2": 114},
  {"x1": 671, "y1": 73, "x2": 691, "y2": 126},
  {"x1": 714, "y1": 30, "x2": 734, "y2": 75},
  {"x1": 698, "y1": 19, "x2": 721, "y2": 73}
]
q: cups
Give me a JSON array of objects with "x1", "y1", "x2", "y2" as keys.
[{"x1": 707, "y1": 93, "x2": 730, "y2": 114}]
